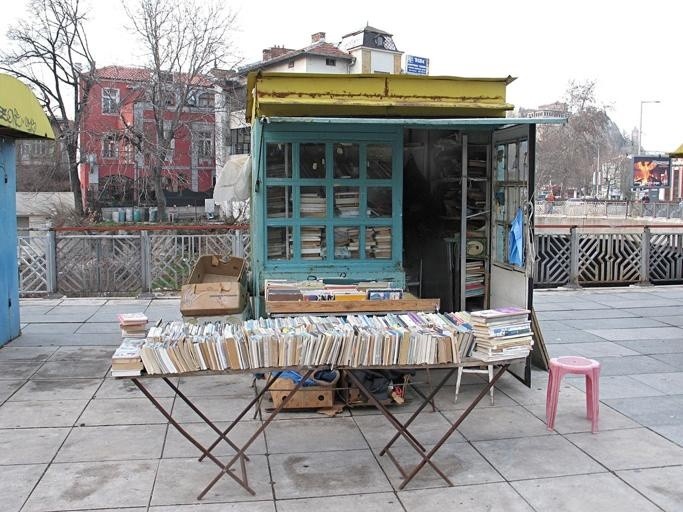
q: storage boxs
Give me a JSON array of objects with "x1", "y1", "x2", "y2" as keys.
[
  {"x1": 267, "y1": 374, "x2": 337, "y2": 411},
  {"x1": 183, "y1": 300, "x2": 252, "y2": 322},
  {"x1": 180, "y1": 282, "x2": 246, "y2": 314},
  {"x1": 187, "y1": 254, "x2": 249, "y2": 289}
]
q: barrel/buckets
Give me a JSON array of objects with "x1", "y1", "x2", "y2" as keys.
[
  {"x1": 149, "y1": 207, "x2": 157, "y2": 222},
  {"x1": 134, "y1": 206, "x2": 141, "y2": 221},
  {"x1": 113, "y1": 211, "x2": 119, "y2": 222},
  {"x1": 120, "y1": 210, "x2": 125, "y2": 221},
  {"x1": 126, "y1": 207, "x2": 133, "y2": 221}
]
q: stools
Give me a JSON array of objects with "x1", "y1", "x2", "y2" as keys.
[{"x1": 545, "y1": 355, "x2": 601, "y2": 435}]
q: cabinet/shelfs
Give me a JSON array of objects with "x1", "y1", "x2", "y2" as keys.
[{"x1": 425, "y1": 123, "x2": 491, "y2": 313}]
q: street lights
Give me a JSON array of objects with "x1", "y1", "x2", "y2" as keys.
[{"x1": 637, "y1": 98, "x2": 664, "y2": 152}]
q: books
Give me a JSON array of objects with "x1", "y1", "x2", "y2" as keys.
[
  {"x1": 112, "y1": 306, "x2": 535, "y2": 379},
  {"x1": 265, "y1": 159, "x2": 486, "y2": 300}
]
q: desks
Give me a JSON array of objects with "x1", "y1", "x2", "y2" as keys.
[
  {"x1": 251, "y1": 284, "x2": 441, "y2": 420},
  {"x1": 115, "y1": 358, "x2": 528, "y2": 501}
]
